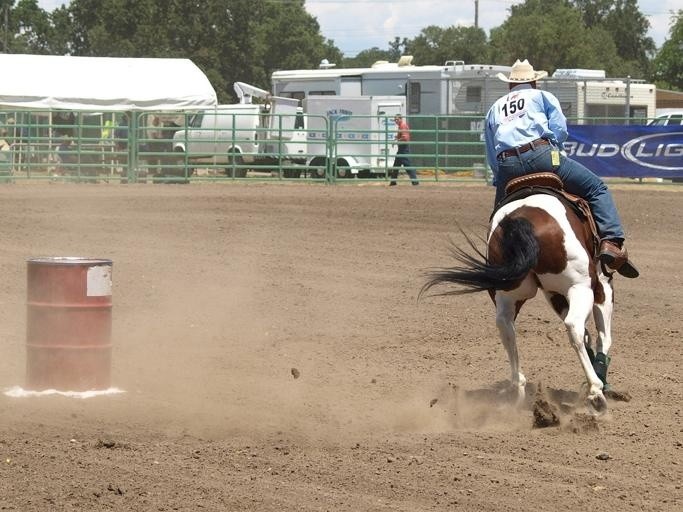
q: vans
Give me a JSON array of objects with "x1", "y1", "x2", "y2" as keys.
[{"x1": 172, "y1": 102, "x2": 307, "y2": 179}]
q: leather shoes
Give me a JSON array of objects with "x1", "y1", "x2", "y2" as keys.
[{"x1": 599, "y1": 240, "x2": 641, "y2": 279}]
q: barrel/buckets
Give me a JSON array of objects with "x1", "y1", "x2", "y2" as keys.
[{"x1": 25, "y1": 255, "x2": 114, "y2": 392}]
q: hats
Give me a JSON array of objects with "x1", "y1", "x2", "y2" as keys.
[{"x1": 498, "y1": 58, "x2": 548, "y2": 84}]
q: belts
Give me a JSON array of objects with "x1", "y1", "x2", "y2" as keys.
[{"x1": 496, "y1": 137, "x2": 550, "y2": 163}]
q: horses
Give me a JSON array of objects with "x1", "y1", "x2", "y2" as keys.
[{"x1": 417, "y1": 184, "x2": 614, "y2": 414}]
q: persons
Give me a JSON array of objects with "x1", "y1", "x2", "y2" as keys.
[
  {"x1": 115, "y1": 111, "x2": 130, "y2": 184},
  {"x1": 388, "y1": 113, "x2": 419, "y2": 186},
  {"x1": 148, "y1": 117, "x2": 161, "y2": 174},
  {"x1": 483, "y1": 59, "x2": 639, "y2": 279}
]
